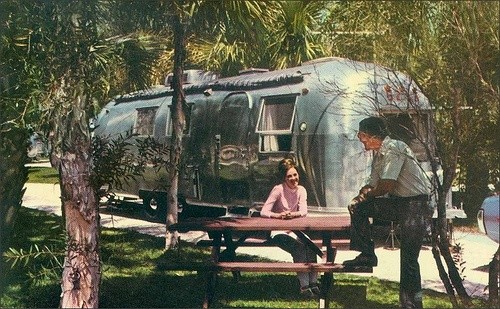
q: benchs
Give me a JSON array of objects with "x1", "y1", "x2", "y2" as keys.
[
  {"x1": 172, "y1": 260, "x2": 372, "y2": 308},
  {"x1": 196, "y1": 239, "x2": 352, "y2": 291}
]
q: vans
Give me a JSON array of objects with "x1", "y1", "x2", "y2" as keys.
[{"x1": 93, "y1": 56, "x2": 468, "y2": 247}]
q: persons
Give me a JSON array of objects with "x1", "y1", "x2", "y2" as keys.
[
  {"x1": 261, "y1": 158, "x2": 320, "y2": 298},
  {"x1": 341, "y1": 115, "x2": 430, "y2": 308}
]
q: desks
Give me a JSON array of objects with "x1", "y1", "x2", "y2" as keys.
[{"x1": 167, "y1": 214, "x2": 374, "y2": 309}]
